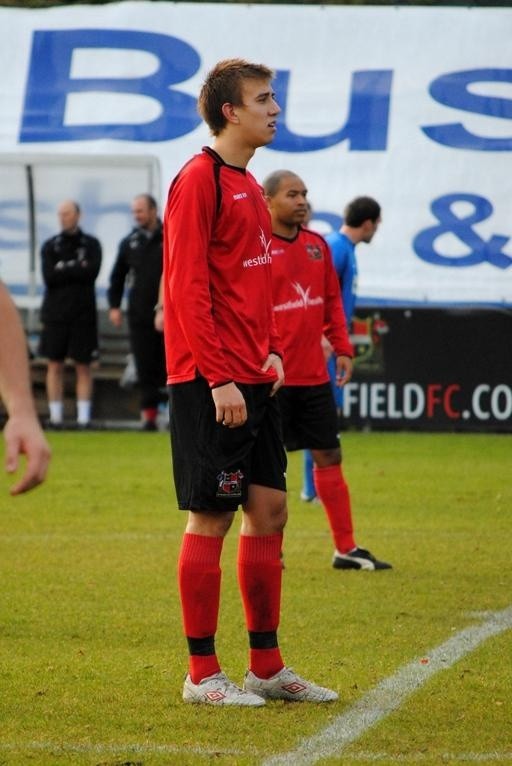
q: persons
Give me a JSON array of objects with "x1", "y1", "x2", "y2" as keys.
[
  {"x1": 106, "y1": 193, "x2": 168, "y2": 431},
  {"x1": 300, "y1": 196, "x2": 381, "y2": 502},
  {"x1": 152, "y1": 272, "x2": 167, "y2": 333},
  {"x1": 302, "y1": 202, "x2": 312, "y2": 225},
  {"x1": 0, "y1": 277, "x2": 51, "y2": 496},
  {"x1": 260, "y1": 169, "x2": 392, "y2": 574},
  {"x1": 39, "y1": 202, "x2": 102, "y2": 429},
  {"x1": 160, "y1": 57, "x2": 340, "y2": 707}
]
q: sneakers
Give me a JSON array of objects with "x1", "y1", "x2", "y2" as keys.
[
  {"x1": 331, "y1": 546, "x2": 395, "y2": 571},
  {"x1": 181, "y1": 665, "x2": 338, "y2": 709}
]
